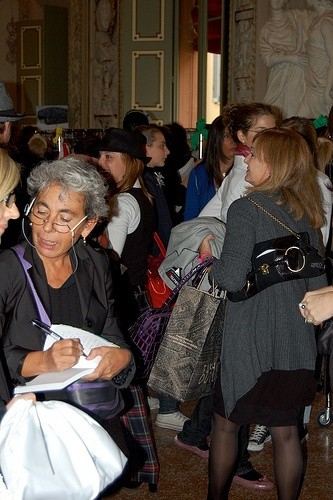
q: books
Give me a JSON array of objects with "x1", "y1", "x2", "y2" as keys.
[{"x1": 13, "y1": 325, "x2": 121, "y2": 392}]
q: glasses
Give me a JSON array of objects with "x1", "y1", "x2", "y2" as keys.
[
  {"x1": 25, "y1": 194, "x2": 90, "y2": 233},
  {"x1": 0, "y1": 193, "x2": 16, "y2": 208}
]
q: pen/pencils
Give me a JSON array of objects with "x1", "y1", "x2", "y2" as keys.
[{"x1": 29, "y1": 318, "x2": 88, "y2": 359}]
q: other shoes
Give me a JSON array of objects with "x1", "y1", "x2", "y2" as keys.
[
  {"x1": 232, "y1": 474, "x2": 275, "y2": 490},
  {"x1": 174, "y1": 436, "x2": 209, "y2": 459}
]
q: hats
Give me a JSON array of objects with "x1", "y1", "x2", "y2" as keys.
[
  {"x1": 94, "y1": 127, "x2": 152, "y2": 164},
  {"x1": 0, "y1": 82, "x2": 25, "y2": 122}
]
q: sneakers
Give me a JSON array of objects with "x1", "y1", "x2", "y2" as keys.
[
  {"x1": 245, "y1": 422, "x2": 272, "y2": 451},
  {"x1": 147, "y1": 397, "x2": 161, "y2": 409},
  {"x1": 155, "y1": 411, "x2": 191, "y2": 431}
]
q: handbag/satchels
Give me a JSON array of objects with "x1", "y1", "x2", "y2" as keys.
[
  {"x1": 64, "y1": 381, "x2": 124, "y2": 420},
  {"x1": 125, "y1": 251, "x2": 226, "y2": 401},
  {"x1": 226, "y1": 197, "x2": 325, "y2": 302}
]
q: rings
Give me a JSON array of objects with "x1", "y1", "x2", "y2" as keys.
[{"x1": 307, "y1": 318, "x2": 313, "y2": 322}]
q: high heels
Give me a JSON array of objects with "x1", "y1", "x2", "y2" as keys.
[{"x1": 123, "y1": 477, "x2": 158, "y2": 493}]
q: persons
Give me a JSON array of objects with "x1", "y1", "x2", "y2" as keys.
[{"x1": 0, "y1": 83, "x2": 333, "y2": 500}]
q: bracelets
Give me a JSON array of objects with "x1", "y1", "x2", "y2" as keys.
[{"x1": 195, "y1": 252, "x2": 210, "y2": 262}]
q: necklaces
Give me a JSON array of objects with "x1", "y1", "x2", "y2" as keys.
[{"x1": 222, "y1": 172, "x2": 226, "y2": 178}]
